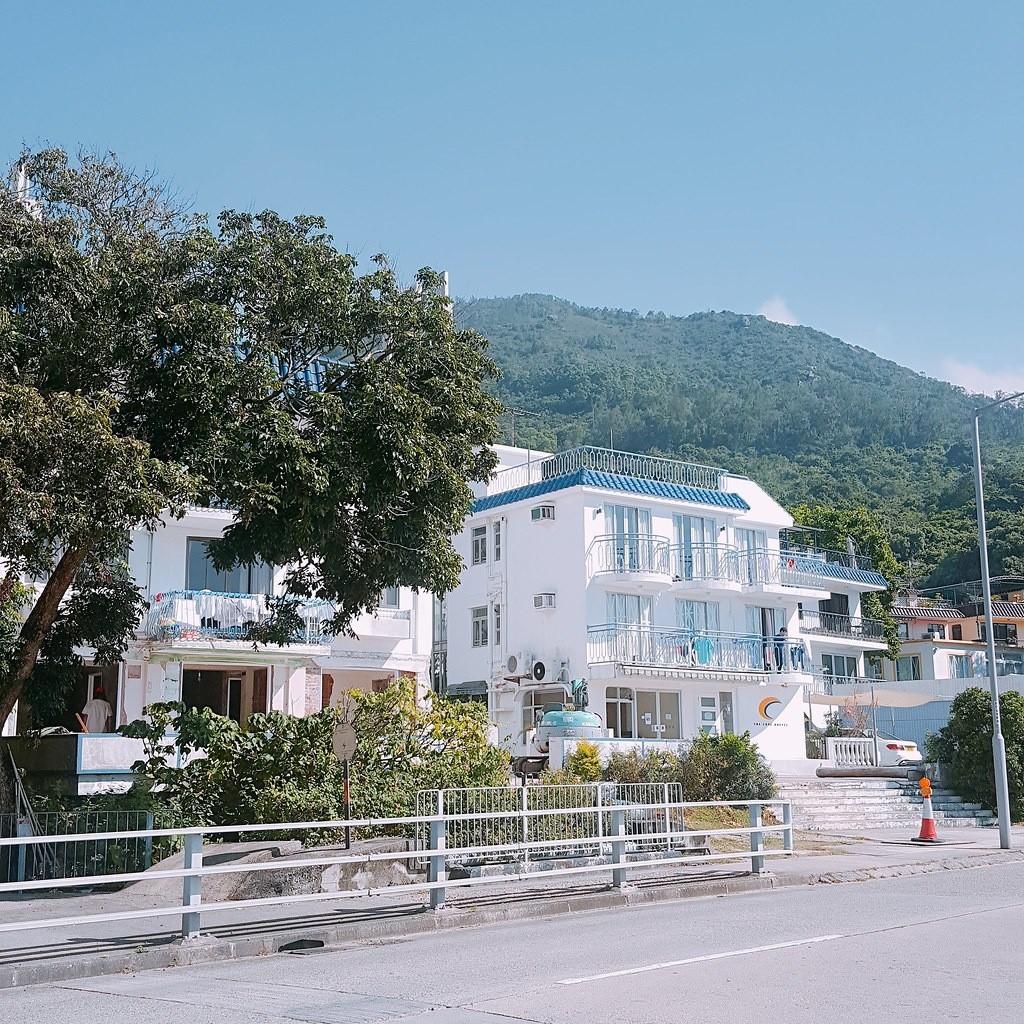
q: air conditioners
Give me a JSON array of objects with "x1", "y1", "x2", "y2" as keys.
[
  {"x1": 503, "y1": 651, "x2": 534, "y2": 681},
  {"x1": 920, "y1": 631, "x2": 940, "y2": 640},
  {"x1": 530, "y1": 660, "x2": 562, "y2": 684}
]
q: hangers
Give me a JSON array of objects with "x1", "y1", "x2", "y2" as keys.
[
  {"x1": 763, "y1": 642, "x2": 804, "y2": 652},
  {"x1": 695, "y1": 634, "x2": 711, "y2": 644}
]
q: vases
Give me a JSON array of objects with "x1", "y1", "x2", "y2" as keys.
[{"x1": 906, "y1": 769, "x2": 925, "y2": 782}]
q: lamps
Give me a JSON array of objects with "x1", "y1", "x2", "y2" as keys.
[
  {"x1": 597, "y1": 504, "x2": 603, "y2": 514},
  {"x1": 720, "y1": 522, "x2": 725, "y2": 532},
  {"x1": 533, "y1": 593, "x2": 557, "y2": 611},
  {"x1": 531, "y1": 505, "x2": 555, "y2": 524}
]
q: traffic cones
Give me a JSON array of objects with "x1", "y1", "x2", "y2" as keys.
[{"x1": 910, "y1": 794, "x2": 946, "y2": 843}]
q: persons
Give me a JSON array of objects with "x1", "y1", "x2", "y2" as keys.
[
  {"x1": 773, "y1": 628, "x2": 786, "y2": 673},
  {"x1": 82, "y1": 686, "x2": 113, "y2": 733}
]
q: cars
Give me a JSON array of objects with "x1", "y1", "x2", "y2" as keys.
[{"x1": 867, "y1": 739, "x2": 923, "y2": 767}]
